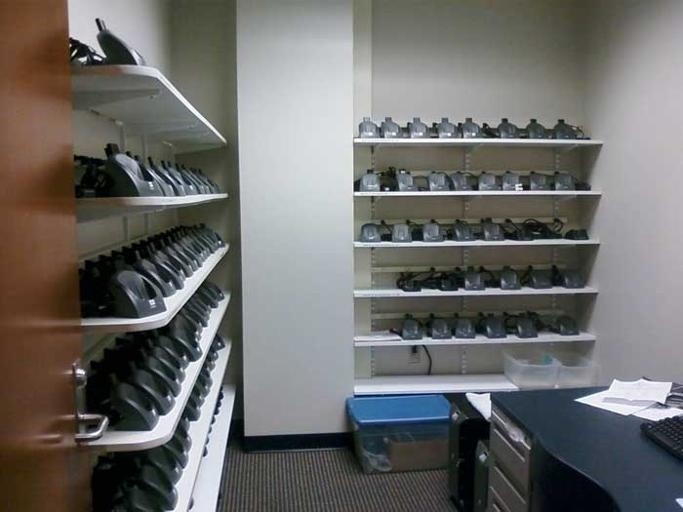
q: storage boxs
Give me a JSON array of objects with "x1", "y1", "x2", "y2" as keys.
[
  {"x1": 345, "y1": 393, "x2": 453, "y2": 472},
  {"x1": 498, "y1": 347, "x2": 560, "y2": 388},
  {"x1": 543, "y1": 346, "x2": 597, "y2": 386}
]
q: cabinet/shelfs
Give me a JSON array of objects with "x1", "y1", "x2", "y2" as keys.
[
  {"x1": 350, "y1": 127, "x2": 601, "y2": 388},
  {"x1": 70, "y1": 61, "x2": 240, "y2": 512}
]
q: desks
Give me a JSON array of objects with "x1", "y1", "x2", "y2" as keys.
[{"x1": 488, "y1": 381, "x2": 683, "y2": 512}]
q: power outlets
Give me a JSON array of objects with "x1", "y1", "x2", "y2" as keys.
[{"x1": 406, "y1": 345, "x2": 420, "y2": 362}]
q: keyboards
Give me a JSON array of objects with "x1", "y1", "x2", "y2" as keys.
[{"x1": 640, "y1": 414, "x2": 683, "y2": 462}]
